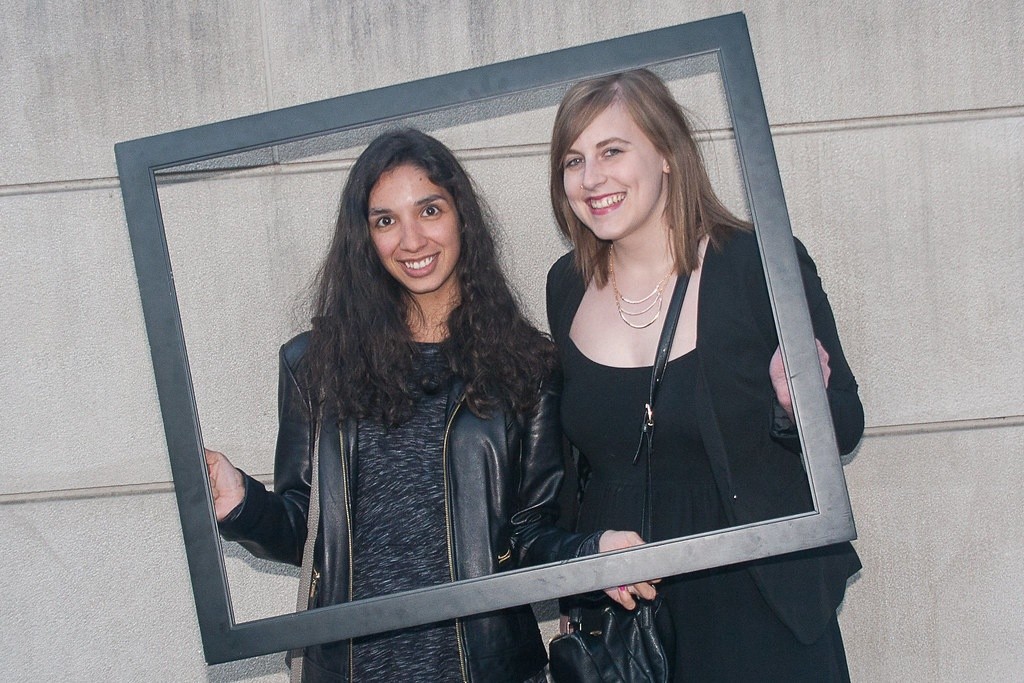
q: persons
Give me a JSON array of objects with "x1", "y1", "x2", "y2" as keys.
[
  {"x1": 203, "y1": 129, "x2": 664, "y2": 683},
  {"x1": 544, "y1": 68, "x2": 865, "y2": 683}
]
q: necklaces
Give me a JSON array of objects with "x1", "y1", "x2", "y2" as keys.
[{"x1": 607, "y1": 240, "x2": 672, "y2": 330}]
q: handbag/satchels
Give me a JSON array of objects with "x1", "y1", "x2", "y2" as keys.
[{"x1": 549, "y1": 528, "x2": 669, "y2": 683}]
